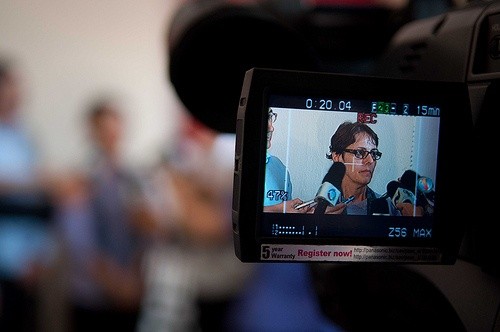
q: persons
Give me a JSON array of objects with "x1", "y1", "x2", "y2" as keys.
[
  {"x1": 0, "y1": 56, "x2": 272, "y2": 331},
  {"x1": 264, "y1": 107, "x2": 315, "y2": 212},
  {"x1": 310, "y1": 121, "x2": 428, "y2": 218}
]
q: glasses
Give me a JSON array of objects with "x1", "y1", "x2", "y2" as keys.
[
  {"x1": 343, "y1": 148, "x2": 382, "y2": 160},
  {"x1": 268, "y1": 113, "x2": 277, "y2": 123}
]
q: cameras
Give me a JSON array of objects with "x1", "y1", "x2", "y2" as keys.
[{"x1": 169, "y1": 0, "x2": 498, "y2": 267}]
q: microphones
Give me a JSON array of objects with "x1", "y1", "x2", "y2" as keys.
[
  {"x1": 386, "y1": 180, "x2": 415, "y2": 215},
  {"x1": 316, "y1": 161, "x2": 345, "y2": 216},
  {"x1": 403, "y1": 169, "x2": 434, "y2": 201}
]
